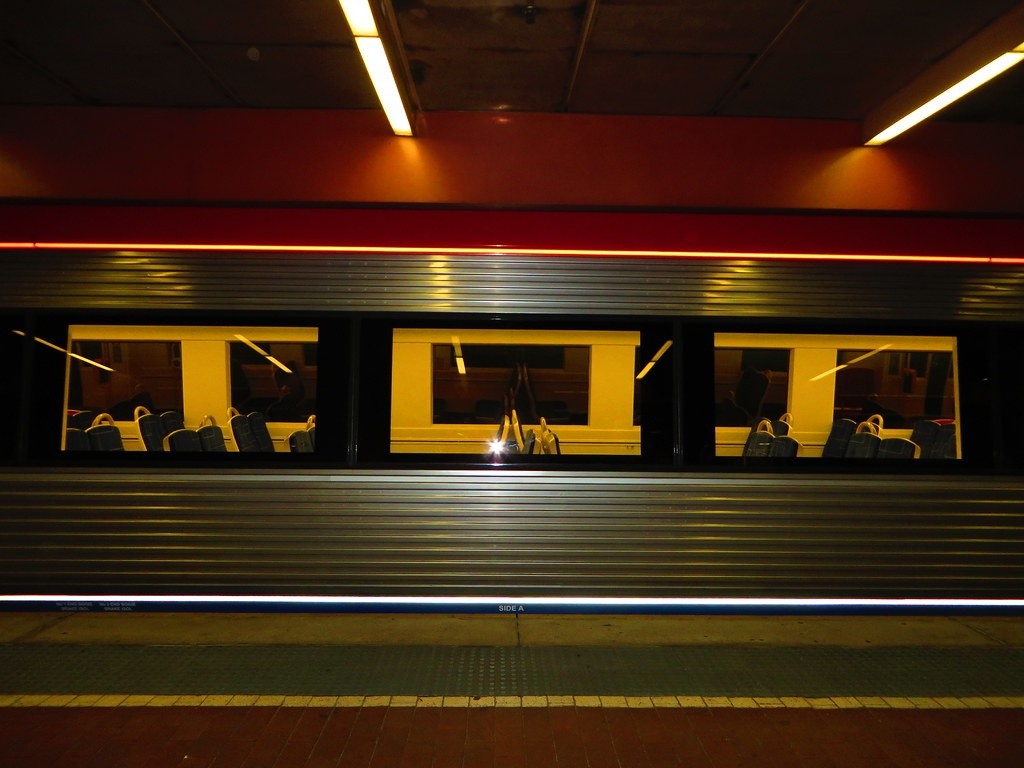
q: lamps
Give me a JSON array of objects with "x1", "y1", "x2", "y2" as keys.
[
  {"x1": 861, "y1": 4, "x2": 1024, "y2": 146},
  {"x1": 338, "y1": 0, "x2": 417, "y2": 136}
]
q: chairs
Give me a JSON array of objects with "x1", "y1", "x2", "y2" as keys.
[
  {"x1": 134, "y1": 406, "x2": 274, "y2": 451},
  {"x1": 821, "y1": 414, "x2": 958, "y2": 458},
  {"x1": 65, "y1": 406, "x2": 124, "y2": 450},
  {"x1": 285, "y1": 414, "x2": 315, "y2": 452},
  {"x1": 741, "y1": 413, "x2": 804, "y2": 457},
  {"x1": 492, "y1": 410, "x2": 561, "y2": 454}
]
